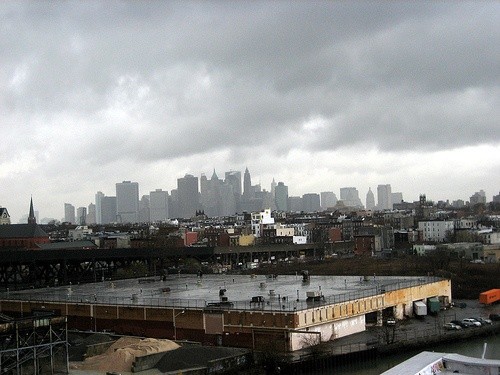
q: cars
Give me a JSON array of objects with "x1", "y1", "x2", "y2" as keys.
[{"x1": 443, "y1": 313, "x2": 500, "y2": 331}]
